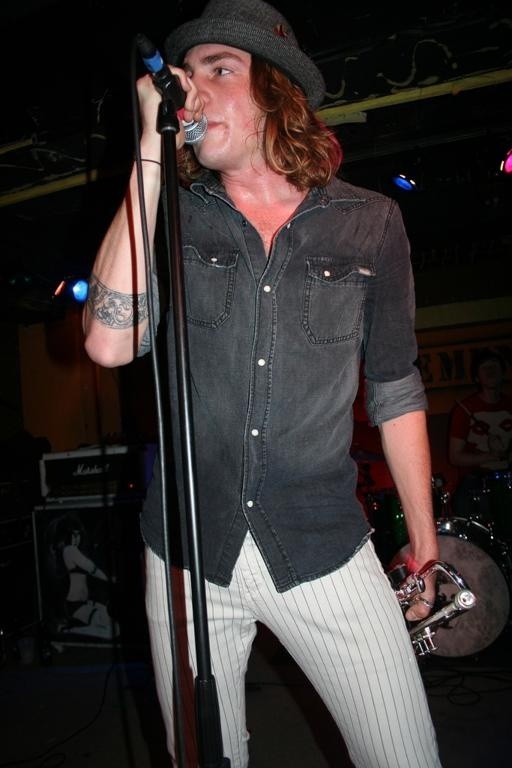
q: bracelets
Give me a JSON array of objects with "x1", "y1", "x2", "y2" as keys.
[{"x1": 128, "y1": 155, "x2": 161, "y2": 167}]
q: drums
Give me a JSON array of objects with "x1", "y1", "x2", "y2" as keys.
[
  {"x1": 364, "y1": 473, "x2": 450, "y2": 567},
  {"x1": 394, "y1": 512, "x2": 512, "y2": 656},
  {"x1": 450, "y1": 469, "x2": 512, "y2": 549}
]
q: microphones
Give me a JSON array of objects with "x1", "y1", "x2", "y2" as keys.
[{"x1": 141, "y1": 38, "x2": 208, "y2": 146}]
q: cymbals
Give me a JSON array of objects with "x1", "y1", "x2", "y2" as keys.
[{"x1": 346, "y1": 450, "x2": 386, "y2": 471}]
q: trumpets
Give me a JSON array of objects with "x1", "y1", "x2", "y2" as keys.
[{"x1": 386, "y1": 559, "x2": 477, "y2": 659}]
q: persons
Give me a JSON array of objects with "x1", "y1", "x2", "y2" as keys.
[
  {"x1": 447, "y1": 350, "x2": 509, "y2": 515},
  {"x1": 59, "y1": 521, "x2": 113, "y2": 628},
  {"x1": 77, "y1": 0, "x2": 441, "y2": 767}
]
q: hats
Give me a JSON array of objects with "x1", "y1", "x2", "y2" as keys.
[{"x1": 164, "y1": 0, "x2": 325, "y2": 108}]
